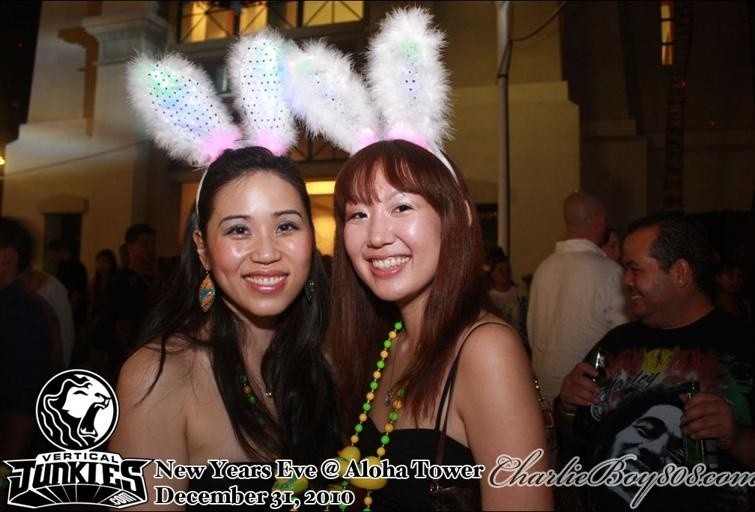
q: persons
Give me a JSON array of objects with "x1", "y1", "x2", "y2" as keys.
[
  {"x1": 330, "y1": 139, "x2": 552, "y2": 512},
  {"x1": 108, "y1": 146, "x2": 333, "y2": 512}
]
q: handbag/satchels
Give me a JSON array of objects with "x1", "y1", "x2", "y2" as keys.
[{"x1": 428, "y1": 322, "x2": 554, "y2": 512}]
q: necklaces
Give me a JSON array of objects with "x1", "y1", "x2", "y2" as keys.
[
  {"x1": 240, "y1": 374, "x2": 309, "y2": 511},
  {"x1": 325, "y1": 321, "x2": 407, "y2": 512}
]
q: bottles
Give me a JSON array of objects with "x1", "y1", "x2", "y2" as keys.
[
  {"x1": 682, "y1": 379, "x2": 720, "y2": 479},
  {"x1": 572, "y1": 344, "x2": 607, "y2": 428}
]
